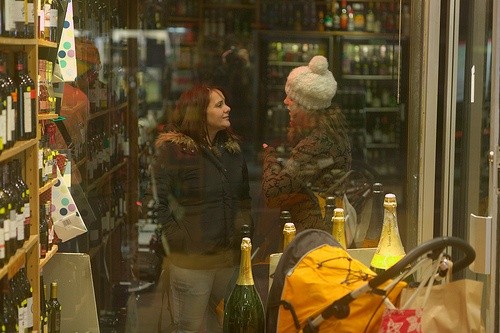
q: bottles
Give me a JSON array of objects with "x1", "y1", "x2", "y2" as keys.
[
  {"x1": 264, "y1": 39, "x2": 395, "y2": 74},
  {"x1": 139, "y1": 107, "x2": 157, "y2": 206},
  {"x1": 264, "y1": 66, "x2": 286, "y2": 106},
  {"x1": 88, "y1": 169, "x2": 127, "y2": 246},
  {"x1": 337, "y1": 80, "x2": 397, "y2": 109},
  {"x1": 264, "y1": 110, "x2": 291, "y2": 146},
  {"x1": 369, "y1": 194, "x2": 415, "y2": 285},
  {"x1": 73, "y1": 0, "x2": 128, "y2": 46},
  {"x1": 0, "y1": 0, "x2": 35, "y2": 39},
  {"x1": 365, "y1": 150, "x2": 401, "y2": 180},
  {"x1": 332, "y1": 208, "x2": 346, "y2": 251},
  {"x1": 341, "y1": 114, "x2": 400, "y2": 144},
  {"x1": 283, "y1": 223, "x2": 296, "y2": 253},
  {"x1": 0, "y1": 120, "x2": 61, "y2": 333},
  {"x1": 280, "y1": 211, "x2": 292, "y2": 253},
  {"x1": 80, "y1": 65, "x2": 128, "y2": 114},
  {"x1": 259, "y1": 0, "x2": 398, "y2": 34},
  {"x1": 364, "y1": 183, "x2": 385, "y2": 248},
  {"x1": 0, "y1": 52, "x2": 59, "y2": 157},
  {"x1": 224, "y1": 225, "x2": 262, "y2": 311},
  {"x1": 323, "y1": 197, "x2": 337, "y2": 235},
  {"x1": 223, "y1": 238, "x2": 266, "y2": 333},
  {"x1": 65, "y1": 113, "x2": 127, "y2": 185},
  {"x1": 139, "y1": 2, "x2": 255, "y2": 103},
  {"x1": 41, "y1": 0, "x2": 61, "y2": 42}
]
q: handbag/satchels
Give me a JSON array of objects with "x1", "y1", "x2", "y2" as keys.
[{"x1": 401, "y1": 260, "x2": 484, "y2": 333}]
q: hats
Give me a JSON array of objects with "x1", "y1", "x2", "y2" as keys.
[{"x1": 284, "y1": 55, "x2": 338, "y2": 110}]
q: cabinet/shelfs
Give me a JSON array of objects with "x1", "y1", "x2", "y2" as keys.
[{"x1": 0, "y1": 1, "x2": 264, "y2": 333}]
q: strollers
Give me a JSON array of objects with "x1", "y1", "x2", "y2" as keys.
[{"x1": 264, "y1": 229, "x2": 477, "y2": 333}]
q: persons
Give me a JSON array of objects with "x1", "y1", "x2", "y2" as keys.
[
  {"x1": 262, "y1": 55, "x2": 351, "y2": 248},
  {"x1": 156, "y1": 85, "x2": 250, "y2": 333},
  {"x1": 216, "y1": 32, "x2": 256, "y2": 107}
]
